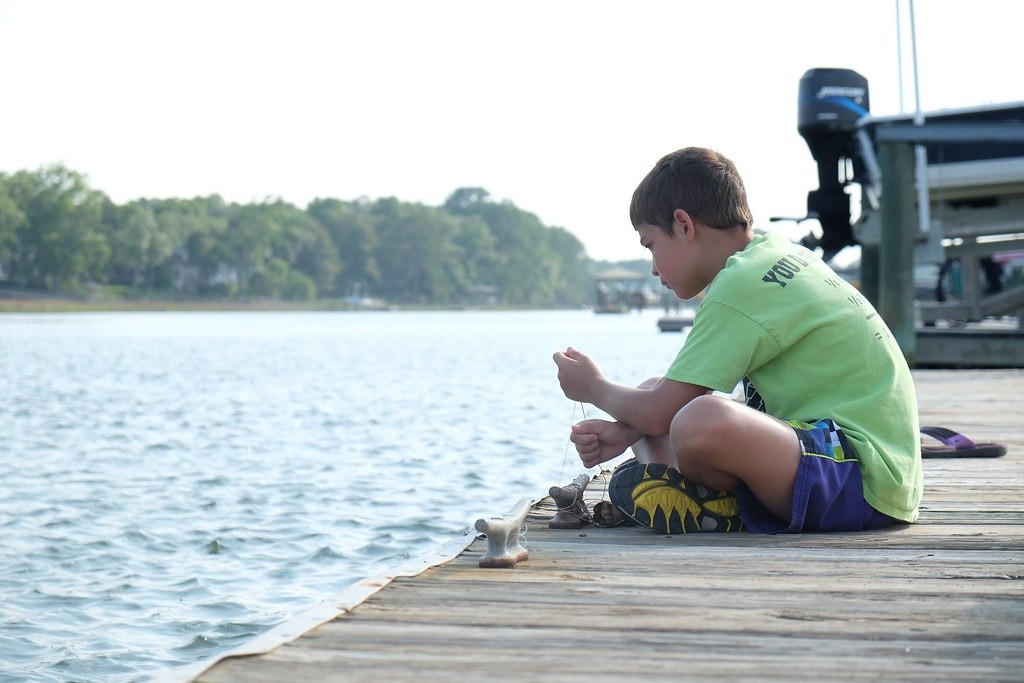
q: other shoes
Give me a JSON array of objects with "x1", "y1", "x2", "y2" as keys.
[{"x1": 609, "y1": 455, "x2": 748, "y2": 535}]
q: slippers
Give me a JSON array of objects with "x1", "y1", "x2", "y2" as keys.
[{"x1": 920, "y1": 426, "x2": 1007, "y2": 457}]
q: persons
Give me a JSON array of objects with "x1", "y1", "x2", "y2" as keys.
[{"x1": 552, "y1": 146, "x2": 923, "y2": 535}]
